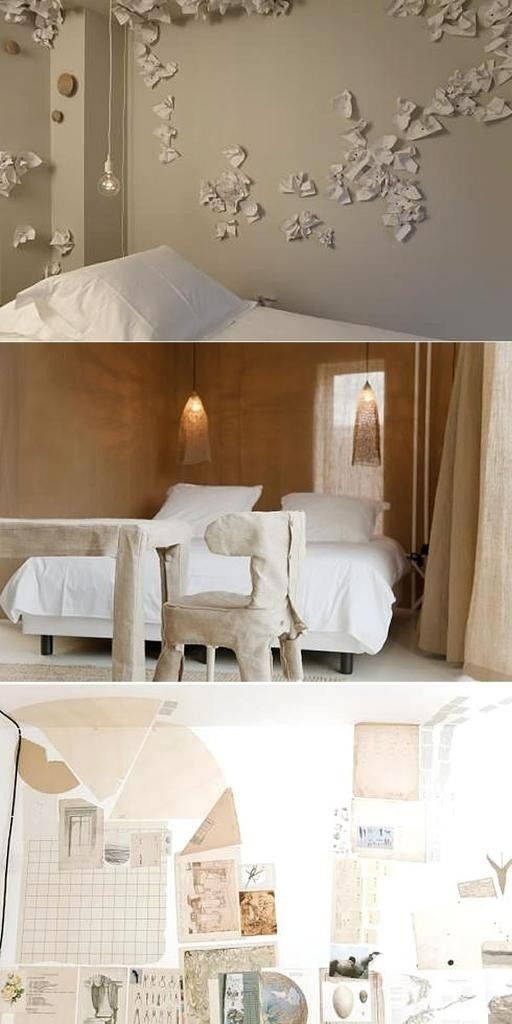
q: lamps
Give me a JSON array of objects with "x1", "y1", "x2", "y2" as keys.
[
  {"x1": 179, "y1": 343, "x2": 212, "y2": 467},
  {"x1": 95, "y1": 0, "x2": 123, "y2": 199},
  {"x1": 351, "y1": 342, "x2": 383, "y2": 468}
]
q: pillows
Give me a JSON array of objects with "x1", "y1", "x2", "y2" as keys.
[
  {"x1": 151, "y1": 481, "x2": 263, "y2": 540},
  {"x1": 15, "y1": 244, "x2": 259, "y2": 343},
  {"x1": 279, "y1": 491, "x2": 386, "y2": 546}
]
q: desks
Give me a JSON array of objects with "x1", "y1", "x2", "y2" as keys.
[{"x1": 0, "y1": 517, "x2": 191, "y2": 681}]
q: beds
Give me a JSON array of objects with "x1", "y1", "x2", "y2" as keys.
[
  {"x1": 0, "y1": 299, "x2": 449, "y2": 342},
  {"x1": 21, "y1": 534, "x2": 401, "y2": 675}
]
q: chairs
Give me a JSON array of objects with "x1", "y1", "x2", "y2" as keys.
[{"x1": 150, "y1": 511, "x2": 308, "y2": 682}]
